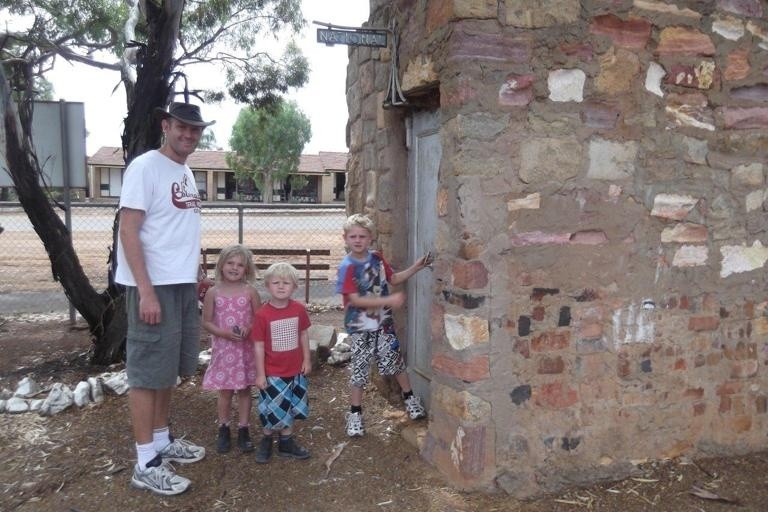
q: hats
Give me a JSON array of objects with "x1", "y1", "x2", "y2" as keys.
[{"x1": 153, "y1": 103, "x2": 216, "y2": 131}]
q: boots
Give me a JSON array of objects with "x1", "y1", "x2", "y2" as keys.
[
  {"x1": 216, "y1": 425, "x2": 232, "y2": 452},
  {"x1": 256, "y1": 437, "x2": 274, "y2": 464},
  {"x1": 278, "y1": 438, "x2": 311, "y2": 459},
  {"x1": 236, "y1": 427, "x2": 256, "y2": 454}
]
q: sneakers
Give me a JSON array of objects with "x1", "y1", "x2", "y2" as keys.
[
  {"x1": 345, "y1": 412, "x2": 364, "y2": 439},
  {"x1": 403, "y1": 393, "x2": 427, "y2": 420},
  {"x1": 131, "y1": 454, "x2": 192, "y2": 496},
  {"x1": 160, "y1": 434, "x2": 205, "y2": 464}
]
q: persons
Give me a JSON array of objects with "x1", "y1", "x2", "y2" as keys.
[
  {"x1": 200, "y1": 242, "x2": 262, "y2": 454},
  {"x1": 332, "y1": 213, "x2": 435, "y2": 438},
  {"x1": 112, "y1": 101, "x2": 218, "y2": 496},
  {"x1": 249, "y1": 261, "x2": 314, "y2": 463}
]
